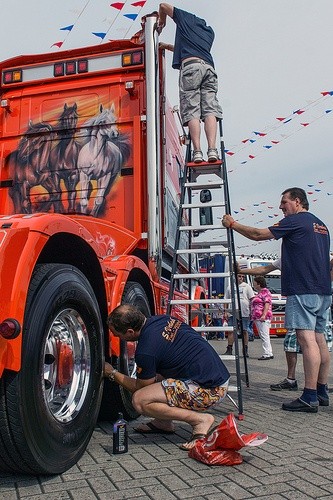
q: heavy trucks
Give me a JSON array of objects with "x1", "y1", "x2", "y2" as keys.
[{"x1": 0, "y1": 14, "x2": 215, "y2": 478}]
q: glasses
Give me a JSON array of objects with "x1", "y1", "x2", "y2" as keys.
[{"x1": 237, "y1": 275, "x2": 244, "y2": 278}]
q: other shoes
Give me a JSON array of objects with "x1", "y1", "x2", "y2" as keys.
[
  {"x1": 282, "y1": 397, "x2": 319, "y2": 413},
  {"x1": 270, "y1": 356, "x2": 274, "y2": 359},
  {"x1": 317, "y1": 393, "x2": 330, "y2": 406},
  {"x1": 207, "y1": 148, "x2": 218, "y2": 161},
  {"x1": 192, "y1": 151, "x2": 203, "y2": 162},
  {"x1": 258, "y1": 356, "x2": 270, "y2": 360}
]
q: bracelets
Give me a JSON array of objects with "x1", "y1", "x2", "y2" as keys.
[
  {"x1": 230, "y1": 221, "x2": 235, "y2": 228},
  {"x1": 109, "y1": 369, "x2": 119, "y2": 378}
]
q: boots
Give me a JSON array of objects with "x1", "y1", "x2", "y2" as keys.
[
  {"x1": 222, "y1": 345, "x2": 232, "y2": 355},
  {"x1": 244, "y1": 345, "x2": 249, "y2": 357}
]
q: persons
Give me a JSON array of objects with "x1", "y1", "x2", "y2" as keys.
[
  {"x1": 234, "y1": 251, "x2": 333, "y2": 390},
  {"x1": 103, "y1": 303, "x2": 231, "y2": 451},
  {"x1": 158, "y1": 2, "x2": 224, "y2": 162},
  {"x1": 221, "y1": 187, "x2": 332, "y2": 412},
  {"x1": 211, "y1": 273, "x2": 275, "y2": 360}
]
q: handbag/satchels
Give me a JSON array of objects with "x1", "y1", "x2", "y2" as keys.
[{"x1": 188, "y1": 412, "x2": 269, "y2": 466}]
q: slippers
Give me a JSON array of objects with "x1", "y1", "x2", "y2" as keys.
[
  {"x1": 179, "y1": 425, "x2": 219, "y2": 451},
  {"x1": 135, "y1": 422, "x2": 175, "y2": 434}
]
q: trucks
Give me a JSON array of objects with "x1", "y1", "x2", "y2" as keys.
[{"x1": 199, "y1": 253, "x2": 288, "y2": 339}]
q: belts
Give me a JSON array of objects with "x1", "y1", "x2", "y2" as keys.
[{"x1": 181, "y1": 59, "x2": 214, "y2": 69}]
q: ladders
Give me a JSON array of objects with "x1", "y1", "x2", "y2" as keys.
[{"x1": 164, "y1": 117, "x2": 252, "y2": 420}]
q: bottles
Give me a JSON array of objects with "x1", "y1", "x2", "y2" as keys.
[{"x1": 113, "y1": 412, "x2": 128, "y2": 454}]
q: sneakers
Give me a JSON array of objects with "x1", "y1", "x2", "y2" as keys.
[
  {"x1": 325, "y1": 383, "x2": 329, "y2": 395},
  {"x1": 270, "y1": 378, "x2": 298, "y2": 391}
]
